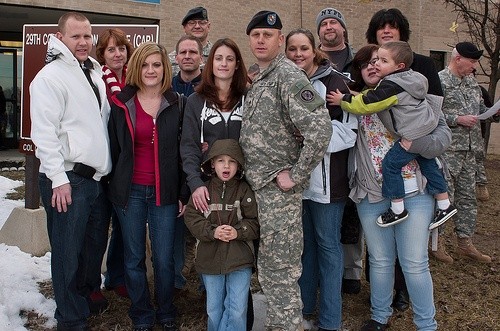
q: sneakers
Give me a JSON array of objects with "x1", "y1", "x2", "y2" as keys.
[
  {"x1": 477, "y1": 185, "x2": 489, "y2": 201},
  {"x1": 376, "y1": 208, "x2": 409, "y2": 227},
  {"x1": 429, "y1": 206, "x2": 458, "y2": 230}
]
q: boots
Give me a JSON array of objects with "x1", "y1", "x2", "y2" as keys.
[
  {"x1": 428, "y1": 236, "x2": 454, "y2": 266},
  {"x1": 456, "y1": 237, "x2": 491, "y2": 263}
]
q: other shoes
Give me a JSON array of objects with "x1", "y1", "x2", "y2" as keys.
[
  {"x1": 89, "y1": 291, "x2": 105, "y2": 301},
  {"x1": 116, "y1": 286, "x2": 130, "y2": 298},
  {"x1": 344, "y1": 279, "x2": 361, "y2": 294},
  {"x1": 363, "y1": 319, "x2": 388, "y2": 331}
]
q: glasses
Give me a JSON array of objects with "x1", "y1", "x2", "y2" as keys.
[{"x1": 358, "y1": 57, "x2": 379, "y2": 70}]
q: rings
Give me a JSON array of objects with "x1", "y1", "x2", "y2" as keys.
[{"x1": 196, "y1": 201, "x2": 198, "y2": 202}]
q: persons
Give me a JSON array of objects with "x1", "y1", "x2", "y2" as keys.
[
  {"x1": 28, "y1": 11, "x2": 113, "y2": 331},
  {"x1": 87, "y1": 29, "x2": 132, "y2": 301},
  {"x1": 184, "y1": 139, "x2": 260, "y2": 331},
  {"x1": 428, "y1": 41, "x2": 500, "y2": 264},
  {"x1": 327, "y1": 42, "x2": 458, "y2": 230},
  {"x1": 471, "y1": 68, "x2": 492, "y2": 200},
  {"x1": 238, "y1": 11, "x2": 333, "y2": 331},
  {"x1": 107, "y1": 41, "x2": 191, "y2": 331},
  {"x1": 347, "y1": 44, "x2": 451, "y2": 331},
  {"x1": 169, "y1": 7, "x2": 215, "y2": 77},
  {"x1": 170, "y1": 35, "x2": 204, "y2": 97},
  {"x1": 316, "y1": 8, "x2": 364, "y2": 294},
  {"x1": 0, "y1": 86, "x2": 8, "y2": 151},
  {"x1": 180, "y1": 39, "x2": 255, "y2": 213},
  {"x1": 342, "y1": 9, "x2": 443, "y2": 312},
  {"x1": 285, "y1": 28, "x2": 358, "y2": 331}
]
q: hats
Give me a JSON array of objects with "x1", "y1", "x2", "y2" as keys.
[
  {"x1": 246, "y1": 10, "x2": 283, "y2": 35},
  {"x1": 182, "y1": 7, "x2": 208, "y2": 26},
  {"x1": 455, "y1": 42, "x2": 484, "y2": 60},
  {"x1": 316, "y1": 7, "x2": 346, "y2": 35}
]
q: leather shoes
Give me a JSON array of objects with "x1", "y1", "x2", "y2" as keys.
[{"x1": 393, "y1": 289, "x2": 410, "y2": 311}]
